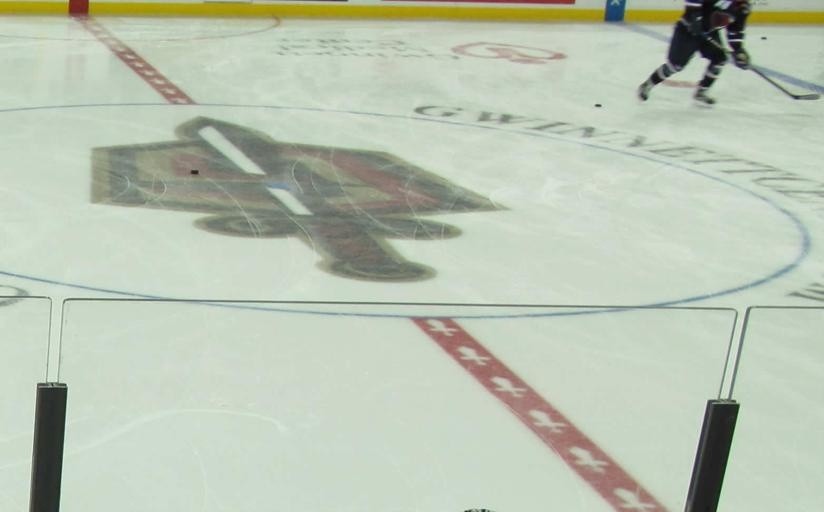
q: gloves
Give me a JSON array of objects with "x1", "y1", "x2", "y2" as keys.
[
  {"x1": 688, "y1": 15, "x2": 702, "y2": 36},
  {"x1": 732, "y1": 47, "x2": 749, "y2": 68}
]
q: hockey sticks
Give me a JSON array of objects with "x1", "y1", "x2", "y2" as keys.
[{"x1": 678, "y1": 16, "x2": 820, "y2": 100}]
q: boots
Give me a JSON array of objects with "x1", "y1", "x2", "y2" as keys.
[
  {"x1": 639, "y1": 78, "x2": 655, "y2": 100},
  {"x1": 694, "y1": 86, "x2": 715, "y2": 104}
]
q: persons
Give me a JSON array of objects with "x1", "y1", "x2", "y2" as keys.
[{"x1": 636, "y1": 0, "x2": 753, "y2": 105}]
